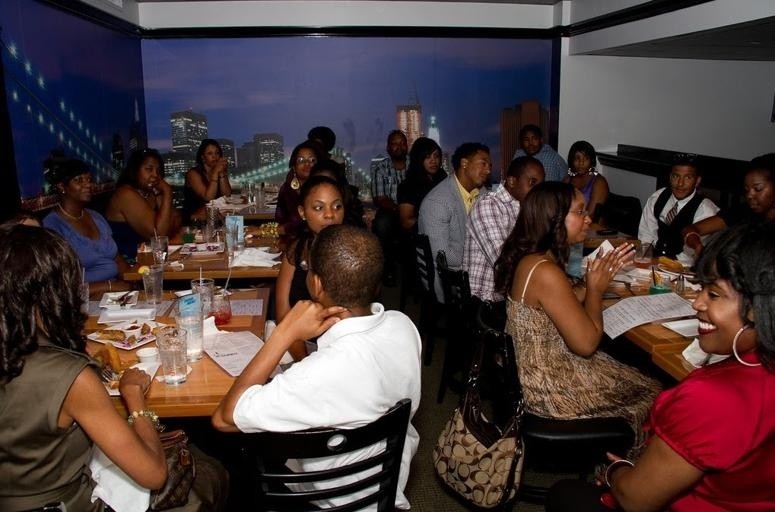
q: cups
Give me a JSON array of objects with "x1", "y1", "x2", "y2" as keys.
[
  {"x1": 156, "y1": 325, "x2": 189, "y2": 386},
  {"x1": 208, "y1": 292, "x2": 232, "y2": 325},
  {"x1": 635, "y1": 243, "x2": 654, "y2": 265},
  {"x1": 142, "y1": 269, "x2": 165, "y2": 305},
  {"x1": 221, "y1": 223, "x2": 240, "y2": 257},
  {"x1": 650, "y1": 274, "x2": 674, "y2": 295},
  {"x1": 181, "y1": 227, "x2": 199, "y2": 243},
  {"x1": 151, "y1": 235, "x2": 169, "y2": 269},
  {"x1": 255, "y1": 189, "x2": 266, "y2": 210},
  {"x1": 206, "y1": 207, "x2": 220, "y2": 242},
  {"x1": 136, "y1": 346, "x2": 160, "y2": 364},
  {"x1": 174, "y1": 309, "x2": 205, "y2": 363},
  {"x1": 190, "y1": 276, "x2": 215, "y2": 319}
]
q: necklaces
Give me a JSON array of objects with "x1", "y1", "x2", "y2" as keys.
[{"x1": 58, "y1": 203, "x2": 84, "y2": 220}]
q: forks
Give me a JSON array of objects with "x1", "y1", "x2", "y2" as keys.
[
  {"x1": 101, "y1": 365, "x2": 117, "y2": 384},
  {"x1": 120, "y1": 294, "x2": 133, "y2": 307},
  {"x1": 117, "y1": 289, "x2": 133, "y2": 302}
]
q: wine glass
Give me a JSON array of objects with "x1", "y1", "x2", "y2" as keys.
[{"x1": 245, "y1": 184, "x2": 255, "y2": 206}]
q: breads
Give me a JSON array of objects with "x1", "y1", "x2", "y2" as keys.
[
  {"x1": 98, "y1": 329, "x2": 126, "y2": 342},
  {"x1": 91, "y1": 342, "x2": 120, "y2": 376},
  {"x1": 660, "y1": 256, "x2": 683, "y2": 270}
]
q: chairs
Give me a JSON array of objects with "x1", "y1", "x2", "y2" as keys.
[
  {"x1": 402, "y1": 232, "x2": 438, "y2": 367},
  {"x1": 435, "y1": 249, "x2": 471, "y2": 402},
  {"x1": 478, "y1": 301, "x2": 635, "y2": 504},
  {"x1": 257, "y1": 399, "x2": 411, "y2": 512}
]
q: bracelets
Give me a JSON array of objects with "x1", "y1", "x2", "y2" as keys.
[
  {"x1": 604, "y1": 459, "x2": 635, "y2": 487},
  {"x1": 127, "y1": 409, "x2": 166, "y2": 434}
]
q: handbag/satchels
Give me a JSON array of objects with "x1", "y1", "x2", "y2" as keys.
[
  {"x1": 146, "y1": 430, "x2": 195, "y2": 512},
  {"x1": 432, "y1": 330, "x2": 527, "y2": 509}
]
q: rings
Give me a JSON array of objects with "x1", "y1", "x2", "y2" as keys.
[{"x1": 609, "y1": 267, "x2": 612, "y2": 271}]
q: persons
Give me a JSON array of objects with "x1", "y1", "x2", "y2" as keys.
[
  {"x1": 545, "y1": 222, "x2": 775, "y2": 512},
  {"x1": 509, "y1": 124, "x2": 568, "y2": 182},
  {"x1": 310, "y1": 158, "x2": 346, "y2": 184},
  {"x1": 743, "y1": 152, "x2": 775, "y2": 226},
  {"x1": 415, "y1": 143, "x2": 492, "y2": 304},
  {"x1": 372, "y1": 131, "x2": 411, "y2": 232},
  {"x1": 562, "y1": 141, "x2": 609, "y2": 218},
  {"x1": 278, "y1": 140, "x2": 323, "y2": 218},
  {"x1": 492, "y1": 181, "x2": 667, "y2": 466},
  {"x1": 106, "y1": 148, "x2": 182, "y2": 259},
  {"x1": 7, "y1": 213, "x2": 43, "y2": 229},
  {"x1": 41, "y1": 149, "x2": 137, "y2": 295},
  {"x1": 210, "y1": 223, "x2": 422, "y2": 512},
  {"x1": 0, "y1": 224, "x2": 230, "y2": 512},
  {"x1": 397, "y1": 137, "x2": 447, "y2": 229},
  {"x1": 638, "y1": 159, "x2": 727, "y2": 258},
  {"x1": 184, "y1": 139, "x2": 232, "y2": 200},
  {"x1": 275, "y1": 176, "x2": 345, "y2": 361},
  {"x1": 464, "y1": 157, "x2": 547, "y2": 305}
]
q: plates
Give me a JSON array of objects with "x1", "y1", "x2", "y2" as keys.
[
  {"x1": 181, "y1": 242, "x2": 226, "y2": 255},
  {"x1": 99, "y1": 292, "x2": 141, "y2": 311},
  {"x1": 175, "y1": 285, "x2": 232, "y2": 302},
  {"x1": 96, "y1": 306, "x2": 156, "y2": 324},
  {"x1": 142, "y1": 244, "x2": 183, "y2": 256},
  {"x1": 101, "y1": 348, "x2": 164, "y2": 397},
  {"x1": 661, "y1": 317, "x2": 704, "y2": 338},
  {"x1": 86, "y1": 317, "x2": 169, "y2": 351}
]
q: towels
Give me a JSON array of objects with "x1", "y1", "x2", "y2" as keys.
[
  {"x1": 209, "y1": 195, "x2": 226, "y2": 205},
  {"x1": 586, "y1": 240, "x2": 614, "y2": 259},
  {"x1": 229, "y1": 248, "x2": 282, "y2": 268},
  {"x1": 89, "y1": 443, "x2": 151, "y2": 512}
]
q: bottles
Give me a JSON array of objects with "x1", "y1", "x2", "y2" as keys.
[{"x1": 678, "y1": 274, "x2": 684, "y2": 292}]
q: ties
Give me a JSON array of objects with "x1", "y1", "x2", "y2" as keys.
[{"x1": 666, "y1": 201, "x2": 679, "y2": 226}]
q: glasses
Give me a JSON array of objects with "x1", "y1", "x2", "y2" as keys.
[
  {"x1": 296, "y1": 157, "x2": 315, "y2": 163},
  {"x1": 569, "y1": 210, "x2": 593, "y2": 217}
]
